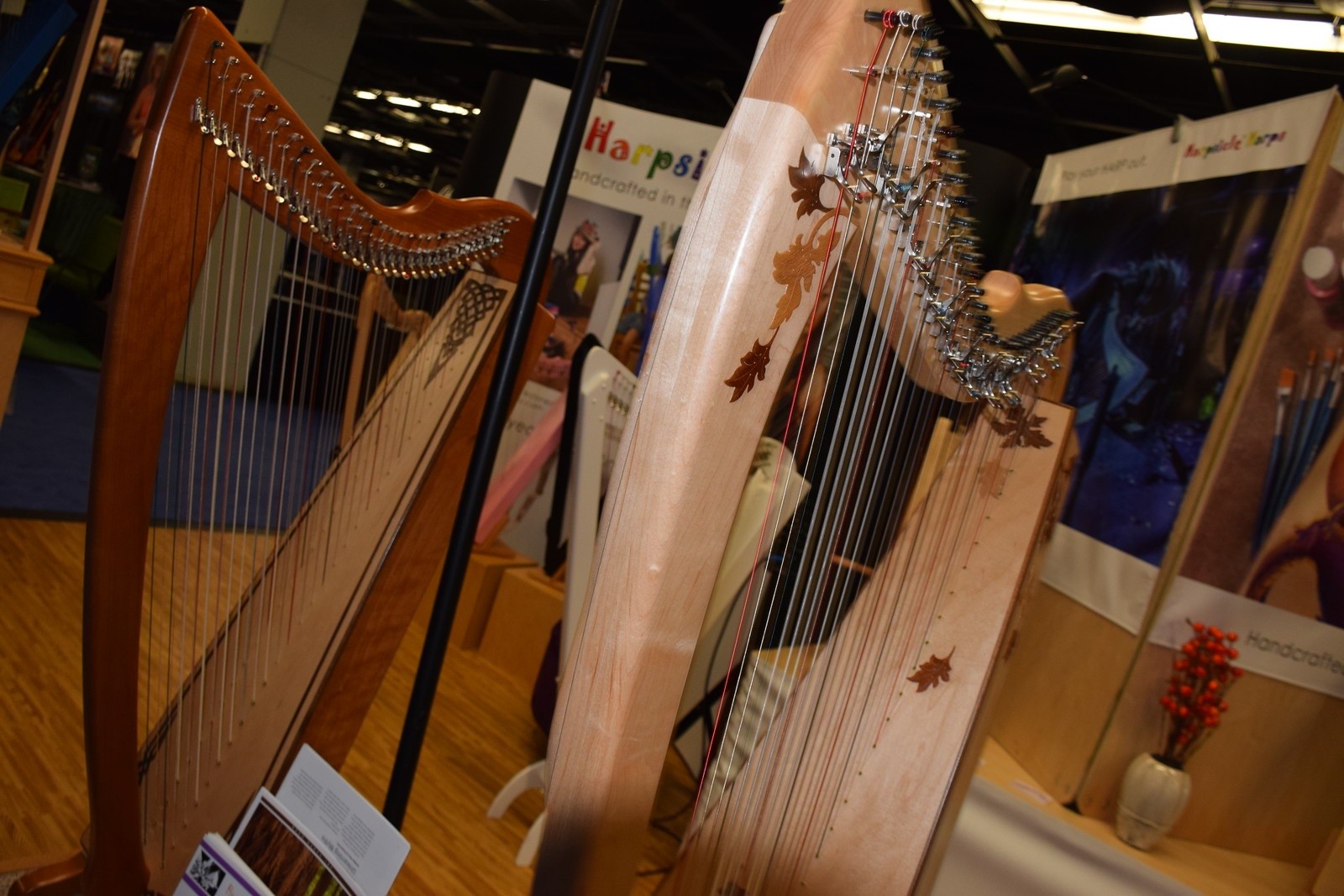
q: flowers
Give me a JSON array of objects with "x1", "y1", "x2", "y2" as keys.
[{"x1": 1158, "y1": 615, "x2": 1241, "y2": 767}]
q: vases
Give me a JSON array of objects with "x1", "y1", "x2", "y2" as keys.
[{"x1": 1117, "y1": 752, "x2": 1191, "y2": 850}]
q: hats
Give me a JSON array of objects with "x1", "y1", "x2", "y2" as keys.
[{"x1": 579, "y1": 219, "x2": 600, "y2": 243}]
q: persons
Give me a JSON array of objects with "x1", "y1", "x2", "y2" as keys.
[
  {"x1": 764, "y1": 262, "x2": 843, "y2": 460},
  {"x1": 636, "y1": 253, "x2": 674, "y2": 377},
  {"x1": 547, "y1": 219, "x2": 600, "y2": 316},
  {"x1": 89, "y1": 55, "x2": 167, "y2": 313}
]
